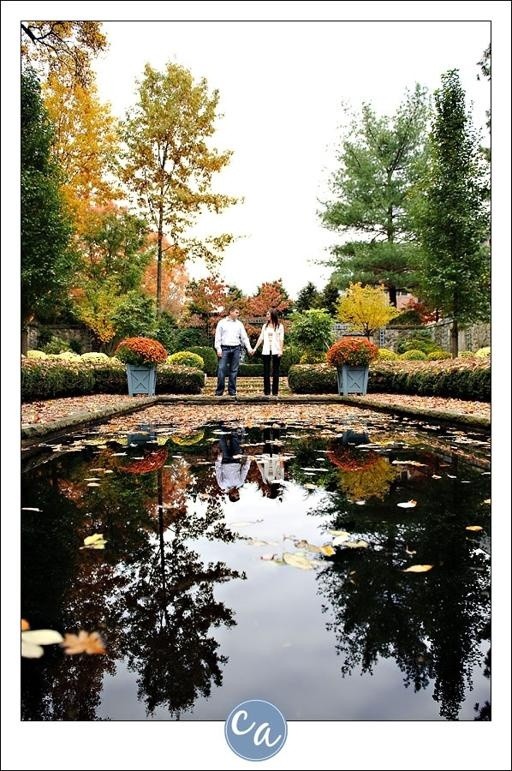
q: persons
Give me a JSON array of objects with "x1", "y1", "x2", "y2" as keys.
[
  {"x1": 214, "y1": 305, "x2": 253, "y2": 396},
  {"x1": 214, "y1": 427, "x2": 252, "y2": 502},
  {"x1": 251, "y1": 307, "x2": 285, "y2": 396},
  {"x1": 248, "y1": 425, "x2": 286, "y2": 499}
]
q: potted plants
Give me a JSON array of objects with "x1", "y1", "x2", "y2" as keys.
[
  {"x1": 112, "y1": 426, "x2": 169, "y2": 475},
  {"x1": 327, "y1": 418, "x2": 380, "y2": 472},
  {"x1": 326, "y1": 335, "x2": 378, "y2": 397},
  {"x1": 116, "y1": 336, "x2": 168, "y2": 396}
]
